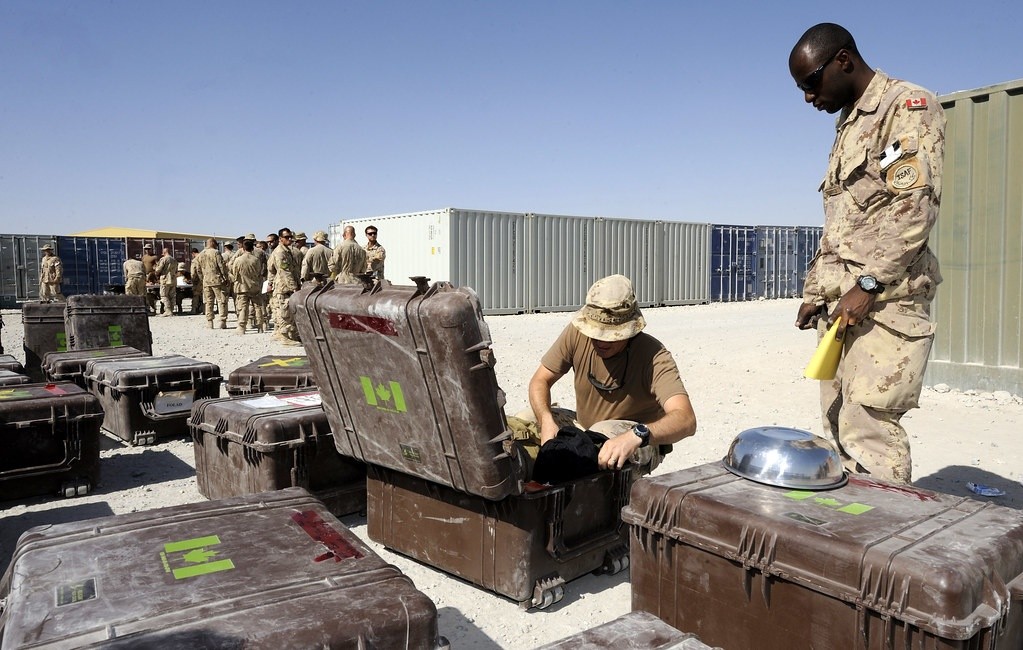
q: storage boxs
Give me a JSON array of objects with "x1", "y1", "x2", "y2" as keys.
[{"x1": 0, "y1": 83, "x2": 1023, "y2": 650}]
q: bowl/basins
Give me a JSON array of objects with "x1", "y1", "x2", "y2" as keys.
[{"x1": 722, "y1": 426, "x2": 849, "y2": 490}]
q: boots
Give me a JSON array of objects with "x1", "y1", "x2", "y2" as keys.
[
  {"x1": 257, "y1": 324, "x2": 263, "y2": 333},
  {"x1": 207, "y1": 320, "x2": 213, "y2": 329},
  {"x1": 264, "y1": 318, "x2": 270, "y2": 330},
  {"x1": 160, "y1": 308, "x2": 172, "y2": 317},
  {"x1": 251, "y1": 319, "x2": 256, "y2": 328},
  {"x1": 220, "y1": 320, "x2": 226, "y2": 328},
  {"x1": 236, "y1": 325, "x2": 245, "y2": 335},
  {"x1": 188, "y1": 307, "x2": 196, "y2": 315},
  {"x1": 277, "y1": 333, "x2": 302, "y2": 346}
]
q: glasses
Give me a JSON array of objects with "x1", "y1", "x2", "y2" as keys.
[
  {"x1": 796, "y1": 44, "x2": 846, "y2": 92},
  {"x1": 267, "y1": 239, "x2": 276, "y2": 243},
  {"x1": 367, "y1": 232, "x2": 377, "y2": 235},
  {"x1": 281, "y1": 235, "x2": 293, "y2": 239},
  {"x1": 587, "y1": 345, "x2": 629, "y2": 392}
]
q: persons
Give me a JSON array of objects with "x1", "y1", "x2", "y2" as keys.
[
  {"x1": 141, "y1": 243, "x2": 166, "y2": 313},
  {"x1": 191, "y1": 226, "x2": 385, "y2": 347},
  {"x1": 515, "y1": 274, "x2": 696, "y2": 481},
  {"x1": 155, "y1": 247, "x2": 178, "y2": 317},
  {"x1": 38, "y1": 243, "x2": 65, "y2": 302},
  {"x1": 123, "y1": 256, "x2": 147, "y2": 296},
  {"x1": 788, "y1": 22, "x2": 947, "y2": 488}
]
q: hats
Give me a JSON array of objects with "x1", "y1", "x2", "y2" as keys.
[
  {"x1": 244, "y1": 234, "x2": 256, "y2": 240},
  {"x1": 224, "y1": 241, "x2": 235, "y2": 246},
  {"x1": 322, "y1": 233, "x2": 329, "y2": 242},
  {"x1": 40, "y1": 244, "x2": 54, "y2": 250},
  {"x1": 296, "y1": 233, "x2": 307, "y2": 240},
  {"x1": 143, "y1": 244, "x2": 153, "y2": 249},
  {"x1": 313, "y1": 231, "x2": 326, "y2": 242},
  {"x1": 570, "y1": 275, "x2": 646, "y2": 343}
]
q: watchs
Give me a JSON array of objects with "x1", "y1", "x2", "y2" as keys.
[
  {"x1": 857, "y1": 275, "x2": 885, "y2": 294},
  {"x1": 631, "y1": 424, "x2": 650, "y2": 448}
]
